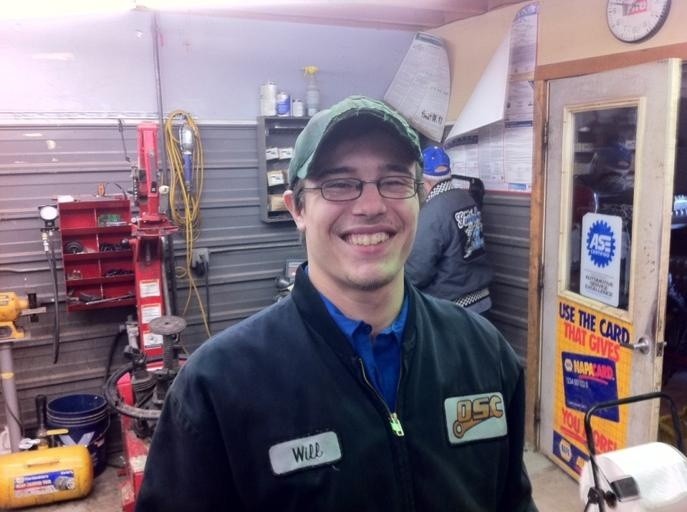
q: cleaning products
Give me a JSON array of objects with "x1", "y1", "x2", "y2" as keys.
[
  {"x1": 277, "y1": 90, "x2": 287, "y2": 118},
  {"x1": 304, "y1": 66, "x2": 320, "y2": 118},
  {"x1": 291, "y1": 97, "x2": 304, "y2": 118}
]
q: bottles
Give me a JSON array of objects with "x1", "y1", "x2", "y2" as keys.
[{"x1": 260, "y1": 80, "x2": 304, "y2": 117}]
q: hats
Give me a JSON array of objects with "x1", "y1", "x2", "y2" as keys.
[
  {"x1": 421, "y1": 145, "x2": 451, "y2": 176},
  {"x1": 286, "y1": 95, "x2": 424, "y2": 191}
]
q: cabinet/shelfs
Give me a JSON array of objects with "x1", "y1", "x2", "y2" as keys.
[
  {"x1": 254, "y1": 114, "x2": 312, "y2": 227},
  {"x1": 56, "y1": 194, "x2": 137, "y2": 316}
]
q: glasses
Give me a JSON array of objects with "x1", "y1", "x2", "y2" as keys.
[{"x1": 303, "y1": 175, "x2": 420, "y2": 202}]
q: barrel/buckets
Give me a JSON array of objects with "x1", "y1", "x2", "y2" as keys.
[{"x1": 46, "y1": 393, "x2": 110, "y2": 477}]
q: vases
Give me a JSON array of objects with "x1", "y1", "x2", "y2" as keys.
[{"x1": 306, "y1": 79, "x2": 320, "y2": 119}]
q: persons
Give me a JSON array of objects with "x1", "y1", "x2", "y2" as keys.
[
  {"x1": 403, "y1": 145, "x2": 494, "y2": 318},
  {"x1": 134, "y1": 95, "x2": 540, "y2": 512}
]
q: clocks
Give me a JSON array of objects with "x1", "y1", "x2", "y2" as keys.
[{"x1": 601, "y1": 0, "x2": 673, "y2": 45}]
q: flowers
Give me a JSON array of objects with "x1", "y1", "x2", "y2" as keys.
[{"x1": 302, "y1": 64, "x2": 318, "y2": 80}]
